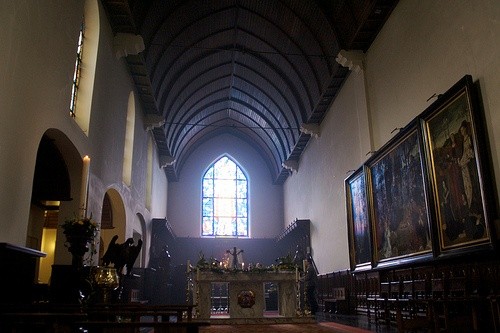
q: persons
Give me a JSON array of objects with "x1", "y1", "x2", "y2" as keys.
[{"x1": 300, "y1": 256, "x2": 322, "y2": 320}]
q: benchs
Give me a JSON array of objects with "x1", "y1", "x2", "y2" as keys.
[{"x1": 322, "y1": 287, "x2": 347, "y2": 314}]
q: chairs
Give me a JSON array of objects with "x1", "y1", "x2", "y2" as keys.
[{"x1": 366, "y1": 269, "x2": 500, "y2": 333}]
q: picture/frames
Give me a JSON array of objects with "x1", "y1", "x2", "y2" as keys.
[{"x1": 342, "y1": 73, "x2": 500, "y2": 274}]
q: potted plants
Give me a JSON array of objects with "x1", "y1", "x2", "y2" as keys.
[{"x1": 59, "y1": 212, "x2": 101, "y2": 256}]
